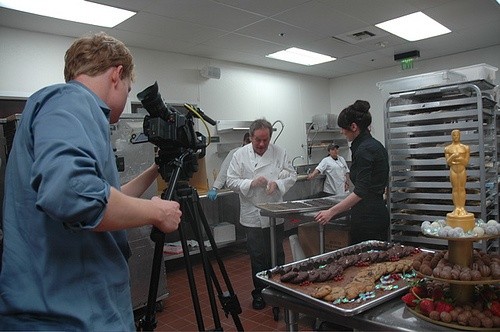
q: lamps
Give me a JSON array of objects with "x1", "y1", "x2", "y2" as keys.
[{"x1": 200, "y1": 66, "x2": 221, "y2": 80}]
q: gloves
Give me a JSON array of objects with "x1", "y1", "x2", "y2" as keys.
[{"x1": 207, "y1": 188, "x2": 217, "y2": 201}]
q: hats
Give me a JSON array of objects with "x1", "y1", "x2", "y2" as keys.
[{"x1": 328, "y1": 144, "x2": 339, "y2": 151}]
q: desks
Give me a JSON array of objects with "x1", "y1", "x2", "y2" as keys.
[
  {"x1": 260, "y1": 209, "x2": 352, "y2": 322},
  {"x1": 261, "y1": 287, "x2": 459, "y2": 332}
]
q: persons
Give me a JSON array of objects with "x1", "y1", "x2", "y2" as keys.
[
  {"x1": 1, "y1": 31, "x2": 182, "y2": 332},
  {"x1": 306, "y1": 144, "x2": 351, "y2": 198},
  {"x1": 227, "y1": 119, "x2": 297, "y2": 309},
  {"x1": 445, "y1": 130, "x2": 470, "y2": 215},
  {"x1": 206, "y1": 133, "x2": 252, "y2": 243},
  {"x1": 314, "y1": 100, "x2": 391, "y2": 246}
]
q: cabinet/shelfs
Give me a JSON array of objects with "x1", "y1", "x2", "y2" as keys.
[
  {"x1": 383, "y1": 84, "x2": 499, "y2": 254},
  {"x1": 164, "y1": 189, "x2": 248, "y2": 261},
  {"x1": 306, "y1": 123, "x2": 348, "y2": 159},
  {"x1": 215, "y1": 120, "x2": 277, "y2": 158}
]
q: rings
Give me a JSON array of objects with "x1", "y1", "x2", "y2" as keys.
[{"x1": 318, "y1": 219, "x2": 322, "y2": 221}]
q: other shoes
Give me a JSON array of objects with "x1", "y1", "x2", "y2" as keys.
[{"x1": 253, "y1": 294, "x2": 265, "y2": 309}]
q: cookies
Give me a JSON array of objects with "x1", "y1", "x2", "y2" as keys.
[{"x1": 279, "y1": 245, "x2": 426, "y2": 302}]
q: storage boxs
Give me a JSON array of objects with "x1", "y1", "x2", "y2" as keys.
[
  {"x1": 312, "y1": 114, "x2": 338, "y2": 129},
  {"x1": 376, "y1": 62, "x2": 499, "y2": 94},
  {"x1": 209, "y1": 221, "x2": 236, "y2": 241}
]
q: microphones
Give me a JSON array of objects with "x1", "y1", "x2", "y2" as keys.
[{"x1": 195, "y1": 108, "x2": 216, "y2": 125}]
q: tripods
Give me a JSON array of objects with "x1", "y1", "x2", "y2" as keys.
[{"x1": 137, "y1": 148, "x2": 245, "y2": 332}]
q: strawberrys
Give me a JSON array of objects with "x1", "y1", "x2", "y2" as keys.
[
  {"x1": 485, "y1": 300, "x2": 500, "y2": 318},
  {"x1": 401, "y1": 280, "x2": 452, "y2": 317}
]
